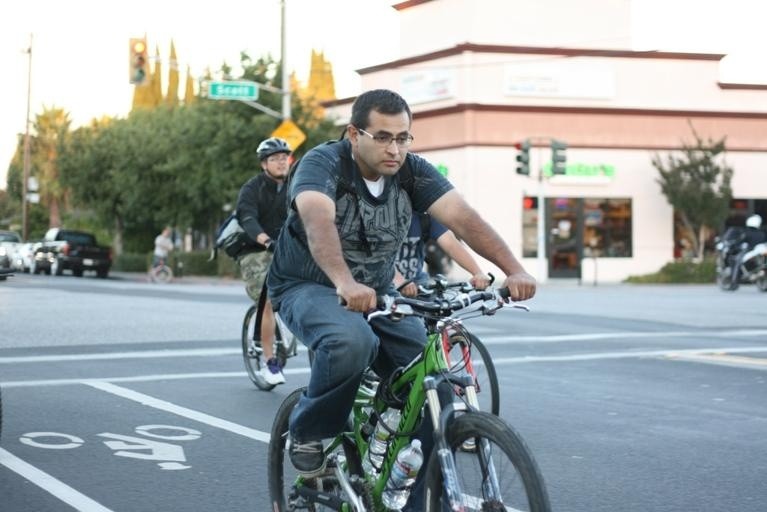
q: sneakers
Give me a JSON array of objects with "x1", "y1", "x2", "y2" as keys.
[
  {"x1": 260, "y1": 357, "x2": 285, "y2": 385},
  {"x1": 288, "y1": 425, "x2": 327, "y2": 478}
]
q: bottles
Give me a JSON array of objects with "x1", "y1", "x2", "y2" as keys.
[
  {"x1": 361, "y1": 406, "x2": 401, "y2": 477},
  {"x1": 381, "y1": 438, "x2": 424, "y2": 510}
]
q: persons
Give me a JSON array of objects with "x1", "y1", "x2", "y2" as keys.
[
  {"x1": 149, "y1": 227, "x2": 174, "y2": 282},
  {"x1": 233, "y1": 136, "x2": 297, "y2": 387},
  {"x1": 724, "y1": 212, "x2": 765, "y2": 288},
  {"x1": 263, "y1": 89, "x2": 535, "y2": 479},
  {"x1": 389, "y1": 211, "x2": 491, "y2": 297}
]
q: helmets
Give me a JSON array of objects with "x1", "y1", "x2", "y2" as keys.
[
  {"x1": 745, "y1": 214, "x2": 763, "y2": 229},
  {"x1": 256, "y1": 137, "x2": 291, "y2": 161}
]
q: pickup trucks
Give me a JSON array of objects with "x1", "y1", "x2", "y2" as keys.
[{"x1": 30, "y1": 227, "x2": 114, "y2": 277}]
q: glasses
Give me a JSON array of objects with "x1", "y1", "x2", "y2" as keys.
[{"x1": 359, "y1": 128, "x2": 414, "y2": 148}]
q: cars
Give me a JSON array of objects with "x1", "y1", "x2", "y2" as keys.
[{"x1": 0, "y1": 228, "x2": 32, "y2": 277}]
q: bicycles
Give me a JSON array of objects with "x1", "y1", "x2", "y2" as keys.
[
  {"x1": 241, "y1": 293, "x2": 299, "y2": 392},
  {"x1": 147, "y1": 244, "x2": 174, "y2": 284},
  {"x1": 267, "y1": 286, "x2": 553, "y2": 512},
  {"x1": 367, "y1": 273, "x2": 501, "y2": 452}
]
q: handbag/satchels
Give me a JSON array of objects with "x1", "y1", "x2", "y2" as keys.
[{"x1": 214, "y1": 210, "x2": 247, "y2": 257}]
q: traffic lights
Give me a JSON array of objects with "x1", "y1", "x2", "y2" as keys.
[
  {"x1": 129, "y1": 37, "x2": 149, "y2": 87},
  {"x1": 550, "y1": 140, "x2": 567, "y2": 175},
  {"x1": 515, "y1": 139, "x2": 530, "y2": 176}
]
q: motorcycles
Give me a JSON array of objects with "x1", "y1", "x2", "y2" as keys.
[{"x1": 712, "y1": 226, "x2": 767, "y2": 292}]
